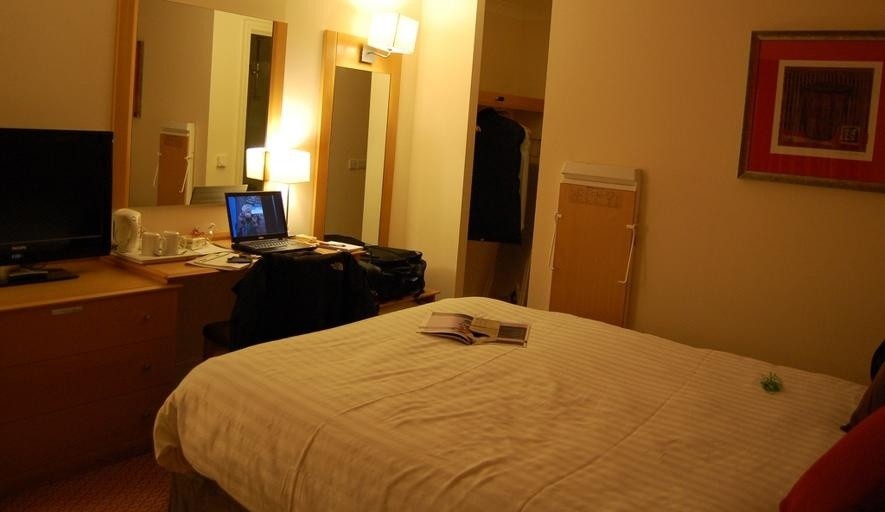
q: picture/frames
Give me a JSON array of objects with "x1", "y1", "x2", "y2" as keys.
[
  {"x1": 133, "y1": 39, "x2": 145, "y2": 119},
  {"x1": 736, "y1": 29, "x2": 885, "y2": 193}
]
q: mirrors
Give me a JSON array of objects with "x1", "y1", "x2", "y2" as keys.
[
  {"x1": 312, "y1": 29, "x2": 401, "y2": 248},
  {"x1": 111, "y1": 0, "x2": 286, "y2": 246}
]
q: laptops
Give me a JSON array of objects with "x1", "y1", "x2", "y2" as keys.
[{"x1": 225, "y1": 191, "x2": 317, "y2": 256}]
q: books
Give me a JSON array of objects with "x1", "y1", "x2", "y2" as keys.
[
  {"x1": 317, "y1": 240, "x2": 364, "y2": 253},
  {"x1": 416, "y1": 310, "x2": 531, "y2": 349},
  {"x1": 186, "y1": 253, "x2": 238, "y2": 273},
  {"x1": 197, "y1": 252, "x2": 262, "y2": 270}
]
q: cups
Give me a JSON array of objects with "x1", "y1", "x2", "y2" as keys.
[
  {"x1": 162, "y1": 230, "x2": 187, "y2": 256},
  {"x1": 140, "y1": 232, "x2": 162, "y2": 256}
]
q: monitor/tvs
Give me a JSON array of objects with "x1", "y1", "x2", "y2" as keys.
[{"x1": 0, "y1": 128, "x2": 114, "y2": 277}]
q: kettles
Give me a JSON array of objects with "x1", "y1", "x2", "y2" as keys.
[{"x1": 112, "y1": 207, "x2": 142, "y2": 254}]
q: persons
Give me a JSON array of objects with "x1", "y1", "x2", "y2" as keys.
[{"x1": 235, "y1": 196, "x2": 261, "y2": 235}]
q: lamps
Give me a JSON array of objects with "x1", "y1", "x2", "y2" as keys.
[
  {"x1": 244, "y1": 146, "x2": 266, "y2": 183},
  {"x1": 270, "y1": 149, "x2": 314, "y2": 232},
  {"x1": 360, "y1": 13, "x2": 420, "y2": 65}
]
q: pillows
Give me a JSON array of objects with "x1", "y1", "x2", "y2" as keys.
[{"x1": 779, "y1": 360, "x2": 885, "y2": 512}]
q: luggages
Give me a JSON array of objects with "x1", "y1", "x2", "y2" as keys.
[{"x1": 351, "y1": 245, "x2": 427, "y2": 301}]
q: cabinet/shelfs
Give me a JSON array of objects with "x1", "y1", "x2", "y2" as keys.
[
  {"x1": 1, "y1": 258, "x2": 184, "y2": 490},
  {"x1": 463, "y1": 89, "x2": 546, "y2": 304},
  {"x1": 110, "y1": 239, "x2": 439, "y2": 382}
]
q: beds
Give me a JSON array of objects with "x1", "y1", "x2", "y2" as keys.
[{"x1": 155, "y1": 296, "x2": 885, "y2": 512}]
